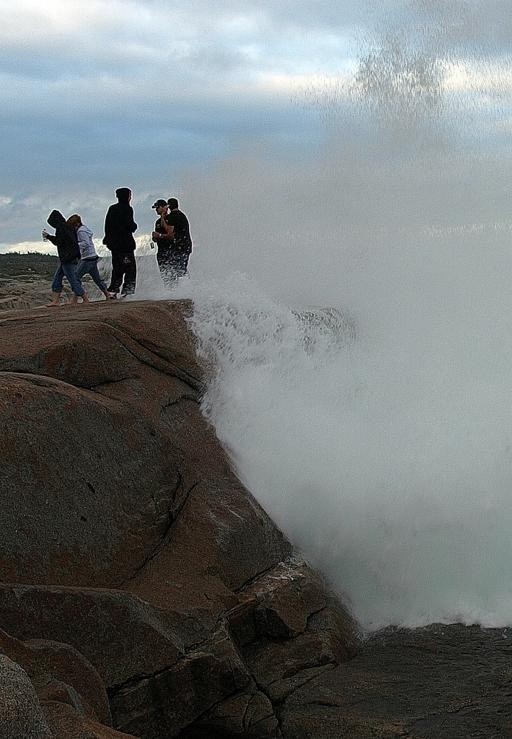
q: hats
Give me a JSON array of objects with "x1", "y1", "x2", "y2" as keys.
[{"x1": 151, "y1": 199, "x2": 167, "y2": 208}]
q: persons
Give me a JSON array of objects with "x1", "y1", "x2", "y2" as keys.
[
  {"x1": 151, "y1": 199, "x2": 179, "y2": 294},
  {"x1": 160, "y1": 197, "x2": 192, "y2": 277},
  {"x1": 64, "y1": 212, "x2": 114, "y2": 304},
  {"x1": 41, "y1": 210, "x2": 90, "y2": 307},
  {"x1": 102, "y1": 188, "x2": 138, "y2": 301}
]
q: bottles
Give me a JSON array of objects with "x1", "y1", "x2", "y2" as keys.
[{"x1": 43, "y1": 229, "x2": 47, "y2": 242}]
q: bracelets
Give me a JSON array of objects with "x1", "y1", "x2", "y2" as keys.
[{"x1": 159, "y1": 233, "x2": 162, "y2": 238}]
